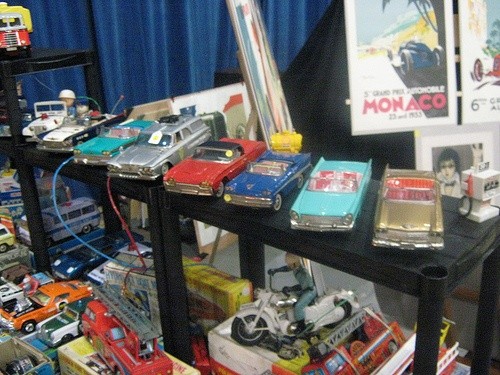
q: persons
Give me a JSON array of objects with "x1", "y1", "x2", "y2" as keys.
[
  {"x1": 268, "y1": 252, "x2": 317, "y2": 333},
  {"x1": 59, "y1": 90, "x2": 90, "y2": 119}
]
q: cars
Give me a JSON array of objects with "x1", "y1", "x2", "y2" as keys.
[
  {"x1": 36, "y1": 111, "x2": 118, "y2": 152},
  {"x1": 370, "y1": 164, "x2": 446, "y2": 252},
  {"x1": 224, "y1": 149, "x2": 313, "y2": 213},
  {"x1": 72, "y1": 120, "x2": 158, "y2": 167},
  {"x1": 163, "y1": 136, "x2": 267, "y2": 199},
  {"x1": 289, "y1": 156, "x2": 373, "y2": 233},
  {"x1": 22, "y1": 100, "x2": 92, "y2": 143}
]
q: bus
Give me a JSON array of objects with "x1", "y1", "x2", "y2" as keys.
[{"x1": 19, "y1": 196, "x2": 100, "y2": 249}]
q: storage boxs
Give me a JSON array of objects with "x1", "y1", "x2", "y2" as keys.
[{"x1": 0, "y1": 82, "x2": 460, "y2": 375}]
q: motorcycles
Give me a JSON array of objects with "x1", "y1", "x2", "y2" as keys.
[{"x1": 232, "y1": 270, "x2": 358, "y2": 350}]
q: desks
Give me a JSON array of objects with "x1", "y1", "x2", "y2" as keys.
[
  {"x1": 0, "y1": 45, "x2": 122, "y2": 274},
  {"x1": 158, "y1": 143, "x2": 499, "y2": 375},
  {"x1": 16, "y1": 115, "x2": 212, "y2": 276}
]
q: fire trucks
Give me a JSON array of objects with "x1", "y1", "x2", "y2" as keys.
[
  {"x1": 302, "y1": 305, "x2": 407, "y2": 375},
  {"x1": 81, "y1": 285, "x2": 174, "y2": 375}
]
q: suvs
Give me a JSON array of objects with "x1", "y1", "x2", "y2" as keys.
[
  {"x1": 0, "y1": 224, "x2": 15, "y2": 253},
  {"x1": 108, "y1": 114, "x2": 213, "y2": 183},
  {"x1": 36, "y1": 298, "x2": 95, "y2": 347}
]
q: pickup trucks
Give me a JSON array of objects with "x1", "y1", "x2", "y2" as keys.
[{"x1": 0, "y1": 279, "x2": 93, "y2": 334}]
